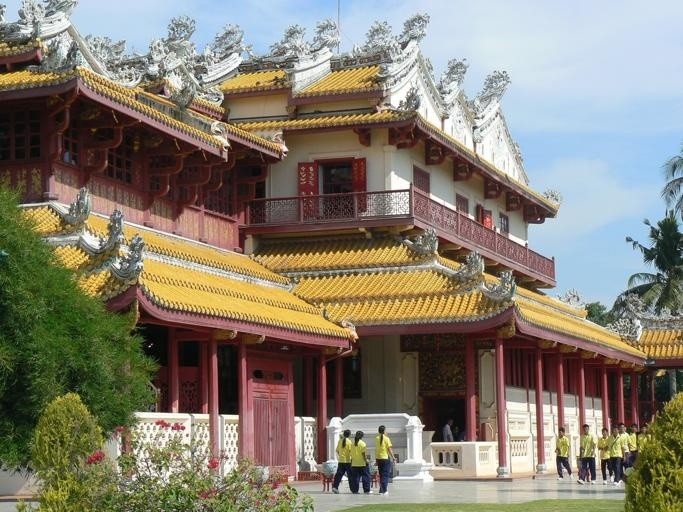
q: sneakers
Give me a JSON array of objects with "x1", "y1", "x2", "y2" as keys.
[
  {"x1": 367, "y1": 491, "x2": 388, "y2": 495},
  {"x1": 332, "y1": 488, "x2": 339, "y2": 494},
  {"x1": 557, "y1": 473, "x2": 623, "y2": 485}
]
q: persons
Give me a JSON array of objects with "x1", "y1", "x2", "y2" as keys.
[
  {"x1": 442, "y1": 418, "x2": 455, "y2": 442},
  {"x1": 332, "y1": 430, "x2": 355, "y2": 494},
  {"x1": 555, "y1": 423, "x2": 651, "y2": 485},
  {"x1": 351, "y1": 431, "x2": 372, "y2": 494},
  {"x1": 375, "y1": 426, "x2": 396, "y2": 495}
]
